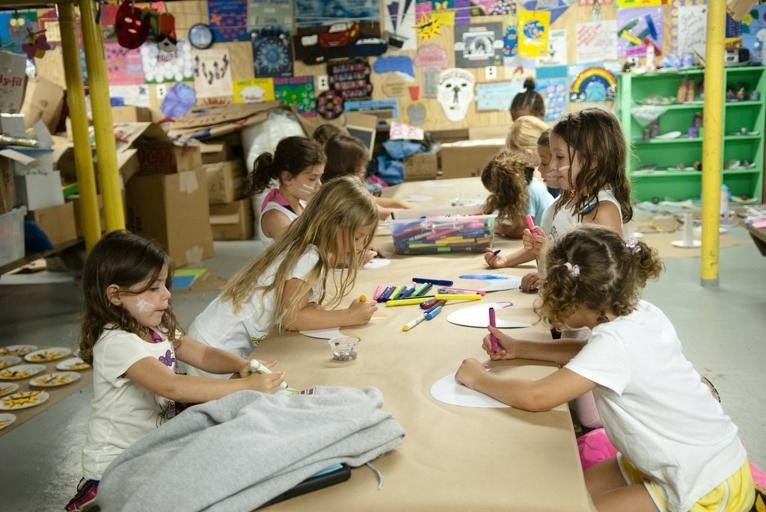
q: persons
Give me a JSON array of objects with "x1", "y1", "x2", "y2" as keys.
[
  {"x1": 321, "y1": 130, "x2": 415, "y2": 222},
  {"x1": 242, "y1": 134, "x2": 375, "y2": 271},
  {"x1": 182, "y1": 174, "x2": 378, "y2": 379},
  {"x1": 478, "y1": 77, "x2": 636, "y2": 439},
  {"x1": 59, "y1": 227, "x2": 285, "y2": 512},
  {"x1": 313, "y1": 122, "x2": 348, "y2": 145},
  {"x1": 451, "y1": 223, "x2": 766, "y2": 511}
]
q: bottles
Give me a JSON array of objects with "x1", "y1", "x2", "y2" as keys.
[
  {"x1": 675, "y1": 79, "x2": 697, "y2": 103},
  {"x1": 694, "y1": 111, "x2": 702, "y2": 125},
  {"x1": 642, "y1": 117, "x2": 660, "y2": 140},
  {"x1": 720, "y1": 183, "x2": 729, "y2": 215},
  {"x1": 645, "y1": 41, "x2": 656, "y2": 72}
]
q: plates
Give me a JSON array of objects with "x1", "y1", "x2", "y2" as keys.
[
  {"x1": 0, "y1": 363, "x2": 47, "y2": 380},
  {"x1": 0, "y1": 413, "x2": 17, "y2": 430},
  {"x1": 55, "y1": 357, "x2": 92, "y2": 371},
  {"x1": 73, "y1": 349, "x2": 80, "y2": 356},
  {"x1": 0, "y1": 381, "x2": 20, "y2": 398},
  {"x1": 24, "y1": 346, "x2": 72, "y2": 363},
  {"x1": 0, "y1": 357, "x2": 22, "y2": 370},
  {"x1": 0, "y1": 391, "x2": 50, "y2": 411},
  {"x1": 0, "y1": 343, "x2": 38, "y2": 357},
  {"x1": 28, "y1": 371, "x2": 81, "y2": 387}
]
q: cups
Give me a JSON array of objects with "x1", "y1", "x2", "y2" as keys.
[
  {"x1": 688, "y1": 128, "x2": 697, "y2": 140},
  {"x1": 326, "y1": 334, "x2": 362, "y2": 361},
  {"x1": 680, "y1": 50, "x2": 694, "y2": 68},
  {"x1": 698, "y1": 127, "x2": 703, "y2": 137}
]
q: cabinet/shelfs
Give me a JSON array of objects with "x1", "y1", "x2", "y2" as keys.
[{"x1": 614, "y1": 63, "x2": 766, "y2": 205}]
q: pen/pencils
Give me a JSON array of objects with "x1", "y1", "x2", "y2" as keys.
[
  {"x1": 494, "y1": 249, "x2": 502, "y2": 256},
  {"x1": 392, "y1": 213, "x2": 492, "y2": 254},
  {"x1": 249, "y1": 358, "x2": 288, "y2": 389},
  {"x1": 372, "y1": 278, "x2": 487, "y2": 331},
  {"x1": 524, "y1": 214, "x2": 538, "y2": 242},
  {"x1": 489, "y1": 307, "x2": 499, "y2": 354},
  {"x1": 359, "y1": 295, "x2": 367, "y2": 303},
  {"x1": 458, "y1": 274, "x2": 506, "y2": 279}
]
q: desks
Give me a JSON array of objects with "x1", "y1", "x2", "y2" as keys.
[{"x1": 228, "y1": 176, "x2": 593, "y2": 512}]
